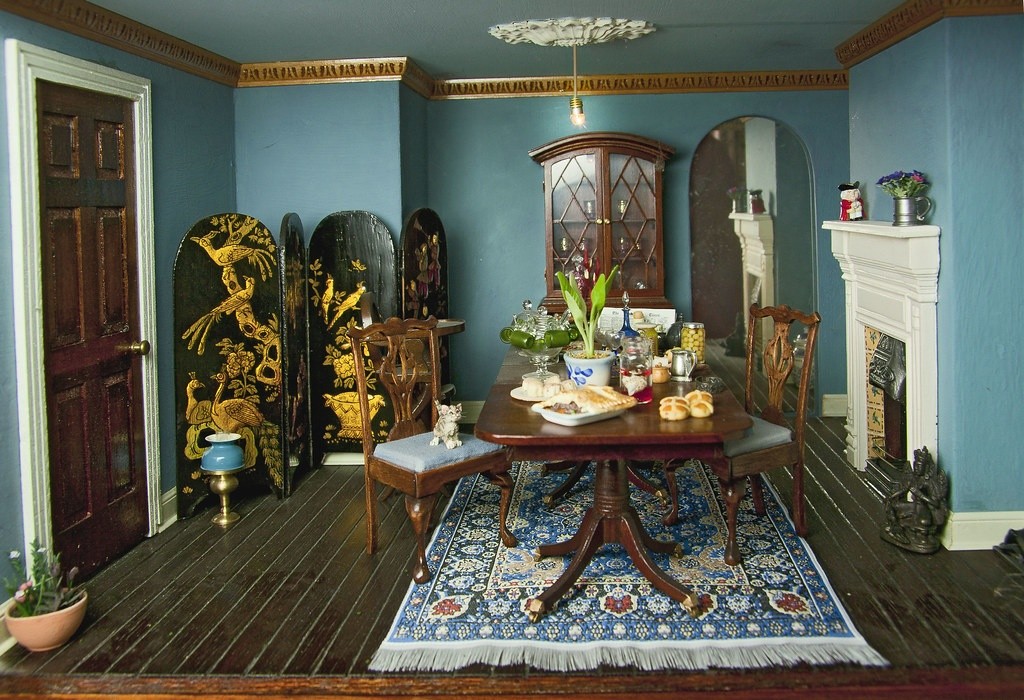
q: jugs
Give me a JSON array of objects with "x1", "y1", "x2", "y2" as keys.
[{"x1": 670, "y1": 350, "x2": 697, "y2": 382}]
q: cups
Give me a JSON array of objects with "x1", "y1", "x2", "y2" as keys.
[
  {"x1": 618, "y1": 237, "x2": 629, "y2": 255},
  {"x1": 559, "y1": 237, "x2": 571, "y2": 254},
  {"x1": 578, "y1": 237, "x2": 590, "y2": 253},
  {"x1": 583, "y1": 200, "x2": 595, "y2": 220},
  {"x1": 617, "y1": 199, "x2": 629, "y2": 219}
]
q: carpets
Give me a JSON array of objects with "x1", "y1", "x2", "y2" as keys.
[{"x1": 367, "y1": 459, "x2": 892, "y2": 671}]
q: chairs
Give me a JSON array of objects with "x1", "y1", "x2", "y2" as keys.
[
  {"x1": 662, "y1": 304, "x2": 822, "y2": 566},
  {"x1": 345, "y1": 314, "x2": 518, "y2": 586}
]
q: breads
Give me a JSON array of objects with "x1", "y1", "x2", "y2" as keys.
[
  {"x1": 544, "y1": 384, "x2": 635, "y2": 413},
  {"x1": 659, "y1": 390, "x2": 715, "y2": 421}
]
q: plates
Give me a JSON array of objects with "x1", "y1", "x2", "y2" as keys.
[
  {"x1": 510, "y1": 387, "x2": 544, "y2": 402},
  {"x1": 531, "y1": 402, "x2": 624, "y2": 426}
]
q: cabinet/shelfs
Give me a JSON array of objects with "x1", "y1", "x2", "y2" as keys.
[{"x1": 527, "y1": 130, "x2": 676, "y2": 313}]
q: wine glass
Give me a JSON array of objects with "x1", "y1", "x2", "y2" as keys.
[{"x1": 594, "y1": 326, "x2": 625, "y2": 372}]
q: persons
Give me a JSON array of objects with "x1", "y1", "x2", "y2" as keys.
[
  {"x1": 838, "y1": 181, "x2": 864, "y2": 221},
  {"x1": 884, "y1": 445, "x2": 940, "y2": 543},
  {"x1": 438, "y1": 404, "x2": 452, "y2": 435}
]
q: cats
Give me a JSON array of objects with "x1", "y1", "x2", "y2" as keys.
[{"x1": 430, "y1": 396, "x2": 462, "y2": 449}]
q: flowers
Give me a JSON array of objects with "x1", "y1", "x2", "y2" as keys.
[
  {"x1": 4, "y1": 535, "x2": 82, "y2": 616},
  {"x1": 726, "y1": 185, "x2": 747, "y2": 199},
  {"x1": 875, "y1": 166, "x2": 930, "y2": 198}
]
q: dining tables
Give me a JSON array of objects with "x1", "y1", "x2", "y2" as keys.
[{"x1": 475, "y1": 343, "x2": 755, "y2": 625}]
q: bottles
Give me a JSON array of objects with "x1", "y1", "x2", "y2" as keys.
[
  {"x1": 620, "y1": 337, "x2": 654, "y2": 403},
  {"x1": 617, "y1": 292, "x2": 640, "y2": 349},
  {"x1": 666, "y1": 312, "x2": 685, "y2": 348},
  {"x1": 635, "y1": 322, "x2": 658, "y2": 356},
  {"x1": 652, "y1": 367, "x2": 668, "y2": 384},
  {"x1": 681, "y1": 322, "x2": 706, "y2": 363}
]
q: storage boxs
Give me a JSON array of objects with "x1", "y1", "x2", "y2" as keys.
[{"x1": 597, "y1": 307, "x2": 678, "y2": 339}]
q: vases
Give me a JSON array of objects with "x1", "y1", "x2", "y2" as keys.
[
  {"x1": 732, "y1": 198, "x2": 744, "y2": 213},
  {"x1": 201, "y1": 433, "x2": 245, "y2": 471},
  {"x1": 892, "y1": 195, "x2": 932, "y2": 227},
  {"x1": 4, "y1": 587, "x2": 88, "y2": 652}
]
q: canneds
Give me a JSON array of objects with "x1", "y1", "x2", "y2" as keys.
[
  {"x1": 680, "y1": 322, "x2": 706, "y2": 364},
  {"x1": 636, "y1": 324, "x2": 658, "y2": 356}
]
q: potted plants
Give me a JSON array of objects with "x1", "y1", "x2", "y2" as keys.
[{"x1": 552, "y1": 264, "x2": 620, "y2": 386}]
